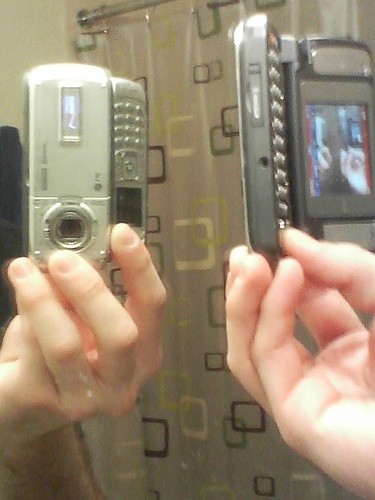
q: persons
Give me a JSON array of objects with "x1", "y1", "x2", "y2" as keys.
[
  {"x1": 223, "y1": 220, "x2": 374, "y2": 499},
  {"x1": 0, "y1": 220, "x2": 168, "y2": 494}
]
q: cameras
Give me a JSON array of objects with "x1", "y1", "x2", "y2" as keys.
[
  {"x1": 27, "y1": 64, "x2": 147, "y2": 272},
  {"x1": 234, "y1": 14, "x2": 374, "y2": 262}
]
